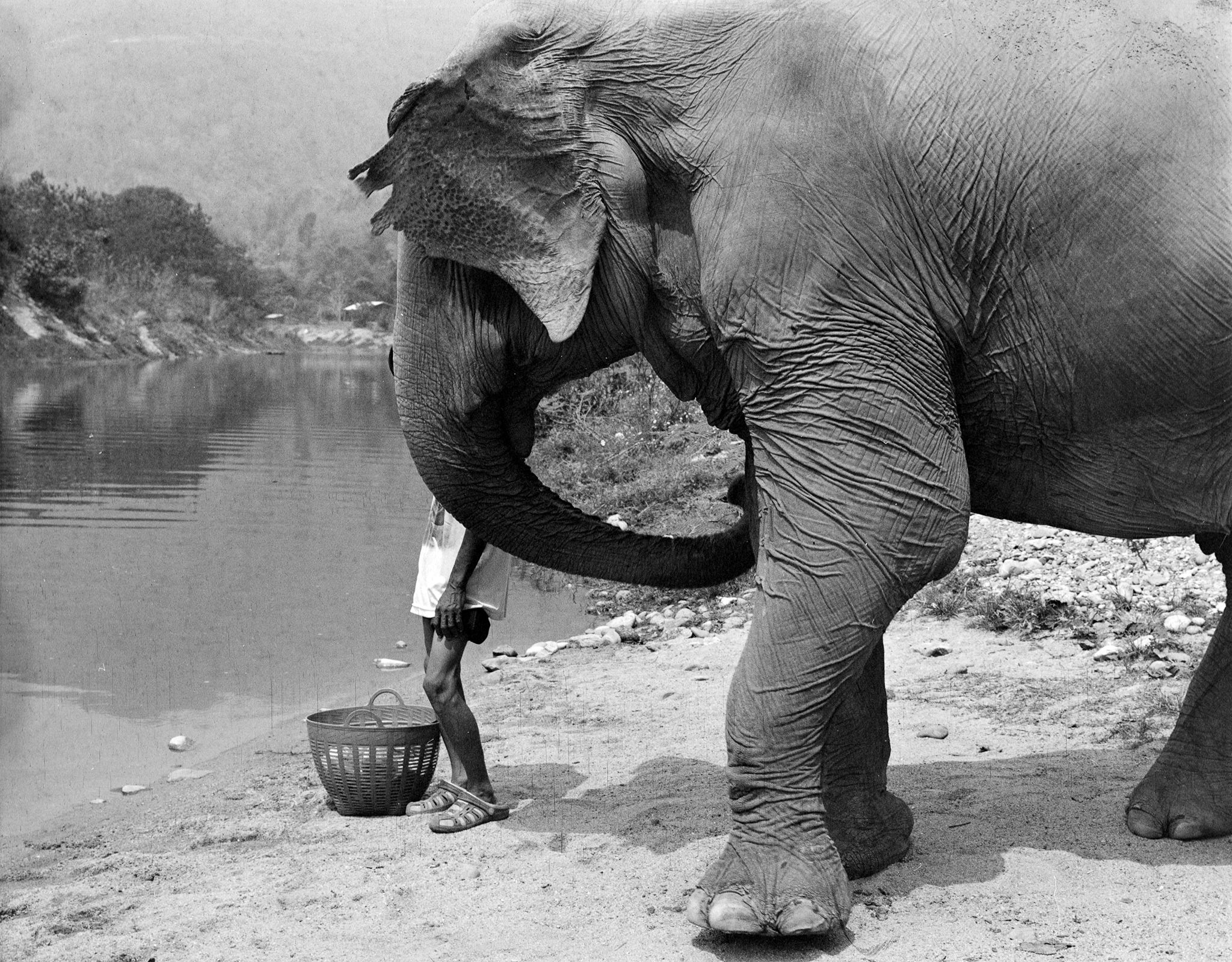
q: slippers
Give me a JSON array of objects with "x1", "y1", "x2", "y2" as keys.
[
  {"x1": 405, "y1": 779, "x2": 467, "y2": 816},
  {"x1": 429, "y1": 790, "x2": 509, "y2": 833}
]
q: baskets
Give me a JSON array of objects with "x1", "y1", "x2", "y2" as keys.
[{"x1": 305, "y1": 688, "x2": 440, "y2": 817}]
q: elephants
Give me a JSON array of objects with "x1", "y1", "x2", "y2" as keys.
[{"x1": 346, "y1": 2, "x2": 1230, "y2": 942}]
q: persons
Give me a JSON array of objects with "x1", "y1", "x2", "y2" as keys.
[{"x1": 388, "y1": 344, "x2": 511, "y2": 833}]
